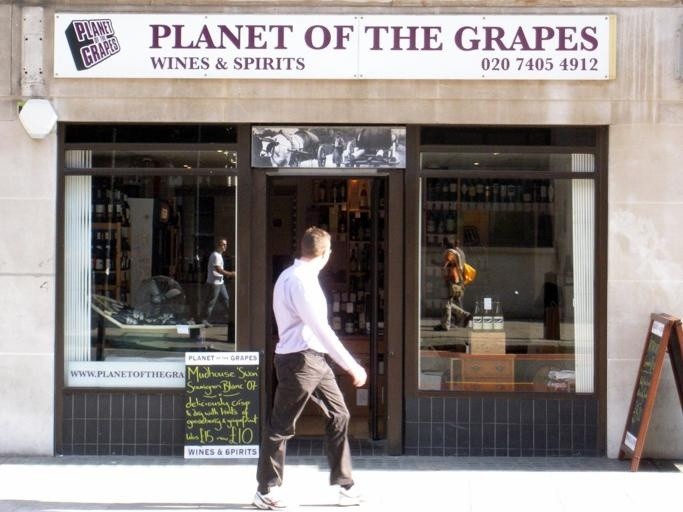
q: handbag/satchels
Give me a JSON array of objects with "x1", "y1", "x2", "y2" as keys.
[{"x1": 463, "y1": 264, "x2": 476, "y2": 285}]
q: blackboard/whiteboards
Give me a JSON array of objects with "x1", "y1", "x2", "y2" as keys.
[
  {"x1": 621, "y1": 313, "x2": 675, "y2": 458},
  {"x1": 184, "y1": 351, "x2": 261, "y2": 459}
]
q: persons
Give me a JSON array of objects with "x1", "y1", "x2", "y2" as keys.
[
  {"x1": 450, "y1": 240, "x2": 465, "y2": 329},
  {"x1": 332, "y1": 131, "x2": 344, "y2": 168},
  {"x1": 430, "y1": 234, "x2": 472, "y2": 332},
  {"x1": 250, "y1": 225, "x2": 367, "y2": 510},
  {"x1": 200, "y1": 236, "x2": 234, "y2": 327}
]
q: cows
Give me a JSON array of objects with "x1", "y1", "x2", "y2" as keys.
[{"x1": 254, "y1": 129, "x2": 319, "y2": 168}]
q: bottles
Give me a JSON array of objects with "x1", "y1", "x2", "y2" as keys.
[
  {"x1": 472, "y1": 301, "x2": 505, "y2": 333},
  {"x1": 426, "y1": 180, "x2": 549, "y2": 202},
  {"x1": 311, "y1": 179, "x2": 387, "y2": 335},
  {"x1": 92, "y1": 190, "x2": 130, "y2": 301},
  {"x1": 426, "y1": 205, "x2": 457, "y2": 247}
]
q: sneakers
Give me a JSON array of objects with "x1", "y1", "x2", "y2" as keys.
[
  {"x1": 338, "y1": 484, "x2": 377, "y2": 507},
  {"x1": 202, "y1": 320, "x2": 213, "y2": 327},
  {"x1": 253, "y1": 491, "x2": 290, "y2": 510},
  {"x1": 433, "y1": 324, "x2": 449, "y2": 331},
  {"x1": 463, "y1": 314, "x2": 473, "y2": 328}
]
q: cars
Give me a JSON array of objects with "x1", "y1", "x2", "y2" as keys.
[{"x1": 91, "y1": 293, "x2": 207, "y2": 350}]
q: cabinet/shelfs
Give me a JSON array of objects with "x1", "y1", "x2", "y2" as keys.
[
  {"x1": 91, "y1": 220, "x2": 132, "y2": 306},
  {"x1": 288, "y1": 169, "x2": 554, "y2": 287}
]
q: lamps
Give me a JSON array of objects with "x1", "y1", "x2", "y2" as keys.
[{"x1": 17, "y1": 98, "x2": 58, "y2": 140}]
